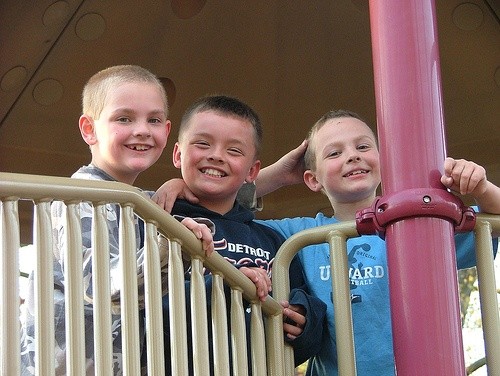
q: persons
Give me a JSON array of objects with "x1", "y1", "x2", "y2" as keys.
[
  {"x1": 137, "y1": 95, "x2": 327, "y2": 376},
  {"x1": 30, "y1": 63, "x2": 309, "y2": 376},
  {"x1": 249, "y1": 108, "x2": 500, "y2": 376}
]
